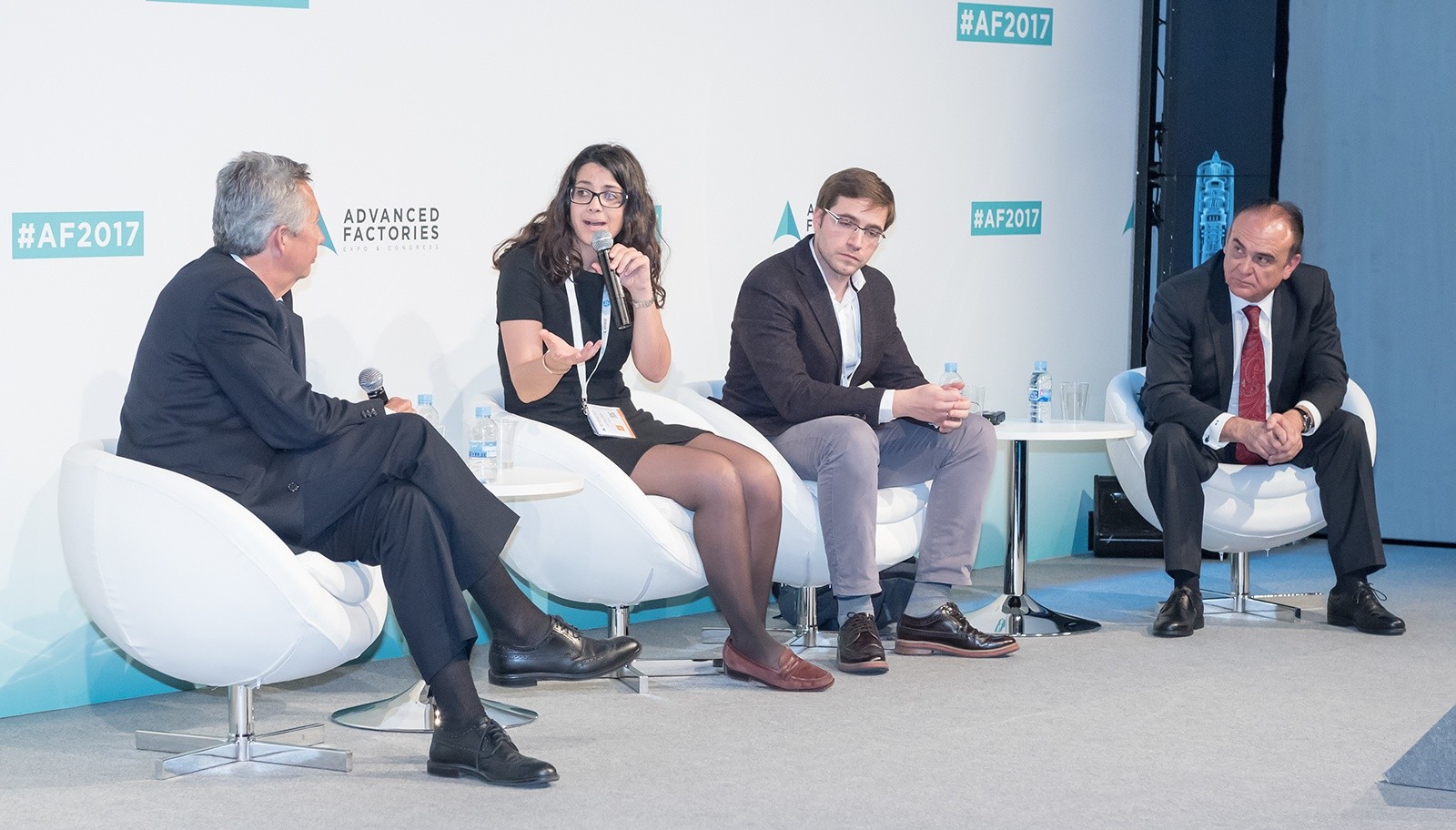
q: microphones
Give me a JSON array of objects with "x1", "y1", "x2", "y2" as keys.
[
  {"x1": 592, "y1": 230, "x2": 632, "y2": 331},
  {"x1": 359, "y1": 368, "x2": 389, "y2": 406}
]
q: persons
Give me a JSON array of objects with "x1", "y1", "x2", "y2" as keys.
[
  {"x1": 716, "y1": 168, "x2": 1023, "y2": 677},
  {"x1": 1140, "y1": 194, "x2": 1405, "y2": 641},
  {"x1": 118, "y1": 150, "x2": 647, "y2": 798},
  {"x1": 493, "y1": 143, "x2": 837, "y2": 698}
]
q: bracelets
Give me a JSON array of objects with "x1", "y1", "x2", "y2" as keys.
[{"x1": 542, "y1": 349, "x2": 572, "y2": 375}]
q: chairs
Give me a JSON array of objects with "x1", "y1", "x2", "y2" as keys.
[
  {"x1": 462, "y1": 383, "x2": 728, "y2": 700},
  {"x1": 54, "y1": 436, "x2": 357, "y2": 784},
  {"x1": 1102, "y1": 363, "x2": 1378, "y2": 627},
  {"x1": 675, "y1": 374, "x2": 938, "y2": 663}
]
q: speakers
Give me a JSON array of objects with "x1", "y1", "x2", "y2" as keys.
[{"x1": 1093, "y1": 474, "x2": 1229, "y2": 560}]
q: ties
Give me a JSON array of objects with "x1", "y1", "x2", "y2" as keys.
[{"x1": 1234, "y1": 305, "x2": 1266, "y2": 464}]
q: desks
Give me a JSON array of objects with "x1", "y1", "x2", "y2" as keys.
[
  {"x1": 958, "y1": 421, "x2": 1145, "y2": 641},
  {"x1": 328, "y1": 467, "x2": 587, "y2": 734}
]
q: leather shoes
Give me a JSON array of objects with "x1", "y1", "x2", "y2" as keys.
[
  {"x1": 893, "y1": 606, "x2": 1019, "y2": 659},
  {"x1": 837, "y1": 616, "x2": 889, "y2": 671},
  {"x1": 488, "y1": 613, "x2": 645, "y2": 687},
  {"x1": 1327, "y1": 585, "x2": 1405, "y2": 633},
  {"x1": 425, "y1": 721, "x2": 559, "y2": 785},
  {"x1": 1152, "y1": 586, "x2": 1205, "y2": 637}
]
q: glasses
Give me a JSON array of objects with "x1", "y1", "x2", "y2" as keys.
[
  {"x1": 819, "y1": 206, "x2": 886, "y2": 245},
  {"x1": 567, "y1": 185, "x2": 629, "y2": 208}
]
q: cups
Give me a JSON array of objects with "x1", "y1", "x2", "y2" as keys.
[
  {"x1": 961, "y1": 385, "x2": 985, "y2": 417},
  {"x1": 1060, "y1": 382, "x2": 1089, "y2": 423},
  {"x1": 494, "y1": 419, "x2": 517, "y2": 470},
  {"x1": 437, "y1": 424, "x2": 444, "y2": 436}
]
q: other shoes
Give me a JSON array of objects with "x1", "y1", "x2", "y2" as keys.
[{"x1": 722, "y1": 628, "x2": 835, "y2": 692}]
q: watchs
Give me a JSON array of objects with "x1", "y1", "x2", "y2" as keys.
[{"x1": 1291, "y1": 405, "x2": 1313, "y2": 432}]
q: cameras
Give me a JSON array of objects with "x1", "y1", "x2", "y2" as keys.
[{"x1": 981, "y1": 410, "x2": 1006, "y2": 425}]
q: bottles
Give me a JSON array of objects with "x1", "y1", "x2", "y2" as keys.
[
  {"x1": 938, "y1": 362, "x2": 964, "y2": 395},
  {"x1": 467, "y1": 406, "x2": 498, "y2": 487},
  {"x1": 1026, "y1": 361, "x2": 1052, "y2": 423},
  {"x1": 416, "y1": 394, "x2": 439, "y2": 430}
]
